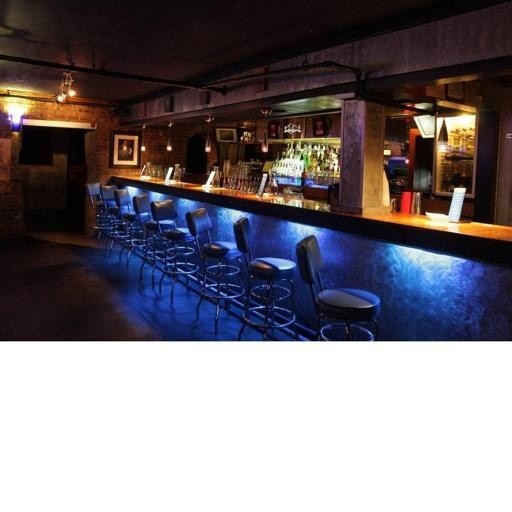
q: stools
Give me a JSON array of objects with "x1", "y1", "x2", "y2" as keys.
[
  {"x1": 186, "y1": 207, "x2": 246, "y2": 336},
  {"x1": 87, "y1": 180, "x2": 174, "y2": 284},
  {"x1": 296, "y1": 234, "x2": 383, "y2": 341},
  {"x1": 231, "y1": 215, "x2": 299, "y2": 340},
  {"x1": 149, "y1": 199, "x2": 199, "y2": 306}
]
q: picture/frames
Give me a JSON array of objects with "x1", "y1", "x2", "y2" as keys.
[
  {"x1": 214, "y1": 126, "x2": 238, "y2": 145},
  {"x1": 431, "y1": 117, "x2": 478, "y2": 200},
  {"x1": 108, "y1": 129, "x2": 142, "y2": 168},
  {"x1": 311, "y1": 115, "x2": 329, "y2": 138}
]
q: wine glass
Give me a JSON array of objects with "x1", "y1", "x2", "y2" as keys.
[{"x1": 149, "y1": 163, "x2": 163, "y2": 178}]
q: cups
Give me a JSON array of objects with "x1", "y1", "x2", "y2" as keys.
[
  {"x1": 411, "y1": 192, "x2": 420, "y2": 215},
  {"x1": 400, "y1": 191, "x2": 411, "y2": 214}
]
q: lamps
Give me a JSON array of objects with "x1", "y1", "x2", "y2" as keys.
[
  {"x1": 436, "y1": 92, "x2": 449, "y2": 148},
  {"x1": 140, "y1": 124, "x2": 146, "y2": 152},
  {"x1": 9, "y1": 109, "x2": 24, "y2": 132},
  {"x1": 258, "y1": 107, "x2": 273, "y2": 154},
  {"x1": 205, "y1": 117, "x2": 215, "y2": 152},
  {"x1": 165, "y1": 120, "x2": 176, "y2": 152},
  {"x1": 54, "y1": 72, "x2": 78, "y2": 103}
]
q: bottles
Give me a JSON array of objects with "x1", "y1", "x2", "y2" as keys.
[
  {"x1": 227, "y1": 159, "x2": 261, "y2": 193},
  {"x1": 267, "y1": 137, "x2": 342, "y2": 195},
  {"x1": 174, "y1": 163, "x2": 180, "y2": 183},
  {"x1": 211, "y1": 166, "x2": 220, "y2": 186}
]
